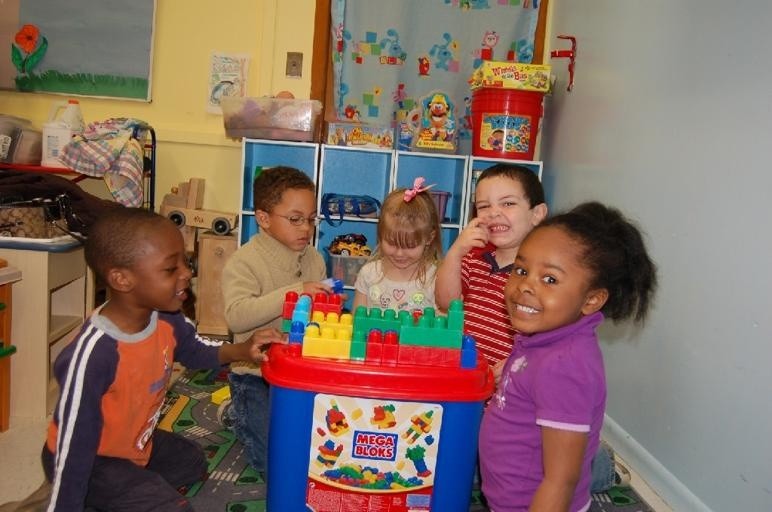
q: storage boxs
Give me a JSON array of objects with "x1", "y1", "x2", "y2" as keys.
[
  {"x1": 258, "y1": 344, "x2": 496, "y2": 511},
  {"x1": 218, "y1": 95, "x2": 328, "y2": 144}
]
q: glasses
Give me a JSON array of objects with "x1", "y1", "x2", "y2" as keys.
[{"x1": 264, "y1": 209, "x2": 321, "y2": 229}]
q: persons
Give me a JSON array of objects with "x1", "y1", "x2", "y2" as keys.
[
  {"x1": 350, "y1": 176, "x2": 445, "y2": 315},
  {"x1": 41, "y1": 206, "x2": 288, "y2": 512},
  {"x1": 434, "y1": 163, "x2": 548, "y2": 413},
  {"x1": 476, "y1": 200, "x2": 659, "y2": 511},
  {"x1": 220, "y1": 167, "x2": 335, "y2": 482}
]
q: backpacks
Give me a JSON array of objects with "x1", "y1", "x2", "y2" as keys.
[{"x1": 322, "y1": 192, "x2": 378, "y2": 214}]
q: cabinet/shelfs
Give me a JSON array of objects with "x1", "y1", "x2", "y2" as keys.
[
  {"x1": 461, "y1": 151, "x2": 545, "y2": 243},
  {"x1": 234, "y1": 135, "x2": 319, "y2": 261},
  {"x1": 390, "y1": 147, "x2": 468, "y2": 258},
  {"x1": 314, "y1": 144, "x2": 394, "y2": 269},
  {"x1": 195, "y1": 229, "x2": 239, "y2": 340},
  {"x1": 0, "y1": 231, "x2": 93, "y2": 504}
]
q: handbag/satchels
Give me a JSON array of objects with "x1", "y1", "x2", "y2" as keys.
[{"x1": 0, "y1": 199, "x2": 67, "y2": 238}]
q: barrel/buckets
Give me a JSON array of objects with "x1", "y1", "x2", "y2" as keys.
[
  {"x1": 471, "y1": 89, "x2": 545, "y2": 161},
  {"x1": 40, "y1": 98, "x2": 84, "y2": 170}
]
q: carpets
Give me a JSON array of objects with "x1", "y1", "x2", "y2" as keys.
[{"x1": 150, "y1": 366, "x2": 654, "y2": 512}]
